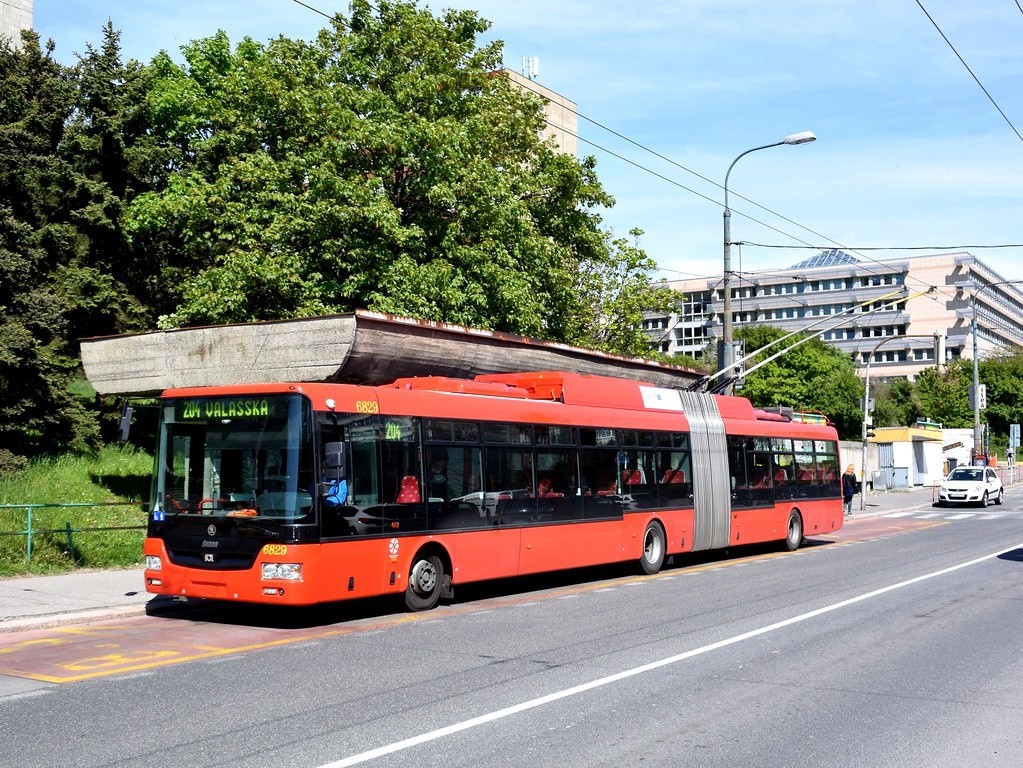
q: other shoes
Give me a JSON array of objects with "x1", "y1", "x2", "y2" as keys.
[{"x1": 848, "y1": 512, "x2": 855, "y2": 516}]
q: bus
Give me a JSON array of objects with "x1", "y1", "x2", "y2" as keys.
[{"x1": 135, "y1": 370, "x2": 847, "y2": 615}]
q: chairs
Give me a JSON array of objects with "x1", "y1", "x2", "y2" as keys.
[{"x1": 395, "y1": 460, "x2": 837, "y2": 504}]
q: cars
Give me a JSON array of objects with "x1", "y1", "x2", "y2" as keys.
[{"x1": 937, "y1": 465, "x2": 1004, "y2": 508}]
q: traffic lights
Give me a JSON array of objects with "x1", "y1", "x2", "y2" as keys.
[{"x1": 865, "y1": 423, "x2": 876, "y2": 444}]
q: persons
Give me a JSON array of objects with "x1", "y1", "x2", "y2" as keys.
[
  {"x1": 428, "y1": 448, "x2": 450, "y2": 498},
  {"x1": 977, "y1": 471, "x2": 989, "y2": 479},
  {"x1": 842, "y1": 464, "x2": 861, "y2": 516},
  {"x1": 1005, "y1": 444, "x2": 1014, "y2": 469},
  {"x1": 300, "y1": 454, "x2": 347, "y2": 525}
]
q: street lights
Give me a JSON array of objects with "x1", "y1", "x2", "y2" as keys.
[{"x1": 722, "y1": 129, "x2": 817, "y2": 399}]
